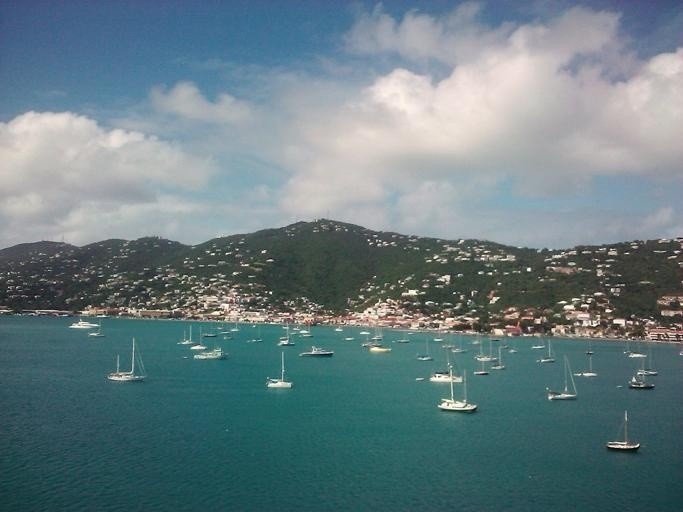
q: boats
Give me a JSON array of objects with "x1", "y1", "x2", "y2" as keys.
[{"x1": 69, "y1": 318, "x2": 101, "y2": 329}]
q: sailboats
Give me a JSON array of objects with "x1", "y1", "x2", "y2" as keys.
[
  {"x1": 87, "y1": 318, "x2": 105, "y2": 337},
  {"x1": 606, "y1": 411, "x2": 640, "y2": 452},
  {"x1": 106, "y1": 337, "x2": 147, "y2": 382}
]
